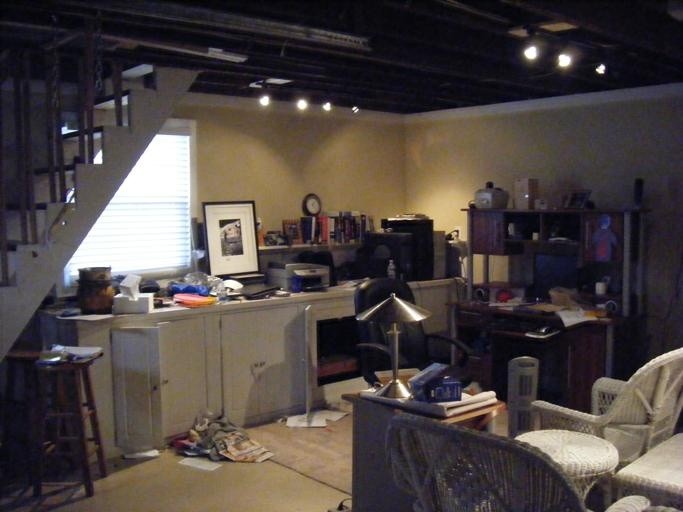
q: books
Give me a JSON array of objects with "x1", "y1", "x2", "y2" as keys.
[{"x1": 299, "y1": 208, "x2": 375, "y2": 246}]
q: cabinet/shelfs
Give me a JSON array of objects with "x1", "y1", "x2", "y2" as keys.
[
  {"x1": 34, "y1": 278, "x2": 466, "y2": 465},
  {"x1": 444, "y1": 207, "x2": 650, "y2": 405}
]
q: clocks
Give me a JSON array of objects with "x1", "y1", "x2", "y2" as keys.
[{"x1": 304, "y1": 193, "x2": 322, "y2": 216}]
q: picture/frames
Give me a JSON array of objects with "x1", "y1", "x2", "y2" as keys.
[{"x1": 202, "y1": 200, "x2": 261, "y2": 278}]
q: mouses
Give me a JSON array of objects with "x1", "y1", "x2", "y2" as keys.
[{"x1": 539, "y1": 325, "x2": 552, "y2": 333}]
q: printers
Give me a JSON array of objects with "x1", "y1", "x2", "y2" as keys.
[{"x1": 265, "y1": 263, "x2": 330, "y2": 293}]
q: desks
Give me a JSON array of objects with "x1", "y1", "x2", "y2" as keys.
[{"x1": 341, "y1": 393, "x2": 506, "y2": 512}]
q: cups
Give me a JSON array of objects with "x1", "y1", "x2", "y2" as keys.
[
  {"x1": 532, "y1": 232, "x2": 539, "y2": 240},
  {"x1": 507, "y1": 223, "x2": 515, "y2": 235},
  {"x1": 594, "y1": 281, "x2": 607, "y2": 294}
]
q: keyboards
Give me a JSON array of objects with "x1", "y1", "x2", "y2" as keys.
[{"x1": 495, "y1": 318, "x2": 535, "y2": 332}]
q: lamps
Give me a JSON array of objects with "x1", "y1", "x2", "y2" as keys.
[
  {"x1": 356, "y1": 293, "x2": 432, "y2": 398},
  {"x1": 258, "y1": 89, "x2": 360, "y2": 114},
  {"x1": 524, "y1": 40, "x2": 611, "y2": 76}
]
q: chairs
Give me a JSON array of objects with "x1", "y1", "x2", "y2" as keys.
[{"x1": 355, "y1": 280, "x2": 469, "y2": 383}]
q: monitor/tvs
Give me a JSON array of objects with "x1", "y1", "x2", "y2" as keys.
[{"x1": 531, "y1": 251, "x2": 578, "y2": 288}]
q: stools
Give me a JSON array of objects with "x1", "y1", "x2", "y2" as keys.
[
  {"x1": 6, "y1": 352, "x2": 41, "y2": 493},
  {"x1": 34, "y1": 357, "x2": 108, "y2": 497}
]
